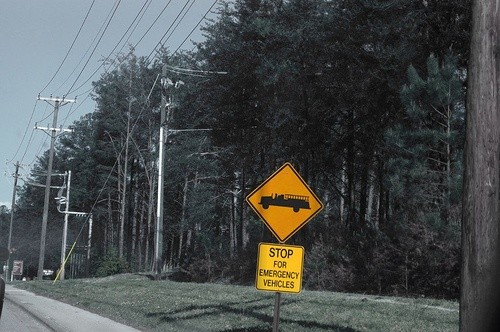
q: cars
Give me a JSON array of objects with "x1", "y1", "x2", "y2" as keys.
[{"x1": 43, "y1": 269, "x2": 55, "y2": 280}]
